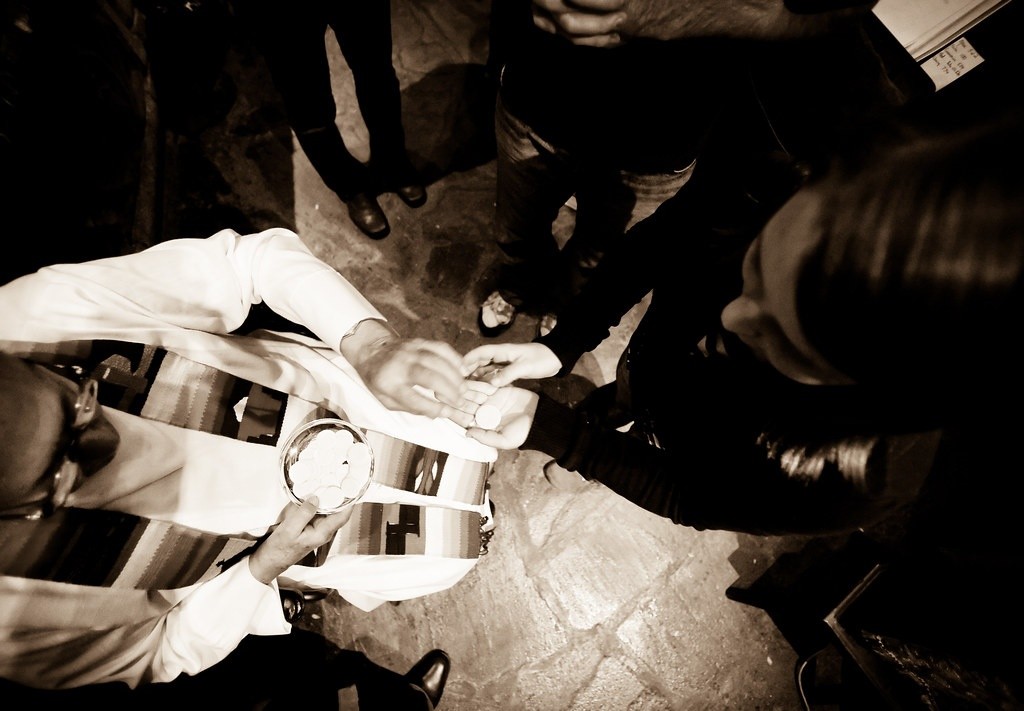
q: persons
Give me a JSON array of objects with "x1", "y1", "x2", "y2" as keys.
[{"x1": 0, "y1": 0, "x2": 1024, "y2": 711}]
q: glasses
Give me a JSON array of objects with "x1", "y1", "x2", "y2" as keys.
[{"x1": 0, "y1": 363, "x2": 99, "y2": 522}]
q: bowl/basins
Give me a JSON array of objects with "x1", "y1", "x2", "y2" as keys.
[{"x1": 278, "y1": 418, "x2": 376, "y2": 514}]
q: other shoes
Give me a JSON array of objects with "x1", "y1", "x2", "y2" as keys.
[
  {"x1": 568, "y1": 379, "x2": 638, "y2": 433},
  {"x1": 478, "y1": 289, "x2": 517, "y2": 337},
  {"x1": 547, "y1": 459, "x2": 599, "y2": 494},
  {"x1": 540, "y1": 308, "x2": 557, "y2": 336}
]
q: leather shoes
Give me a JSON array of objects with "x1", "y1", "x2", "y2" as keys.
[
  {"x1": 389, "y1": 169, "x2": 426, "y2": 208},
  {"x1": 402, "y1": 649, "x2": 451, "y2": 709},
  {"x1": 347, "y1": 190, "x2": 389, "y2": 238}
]
